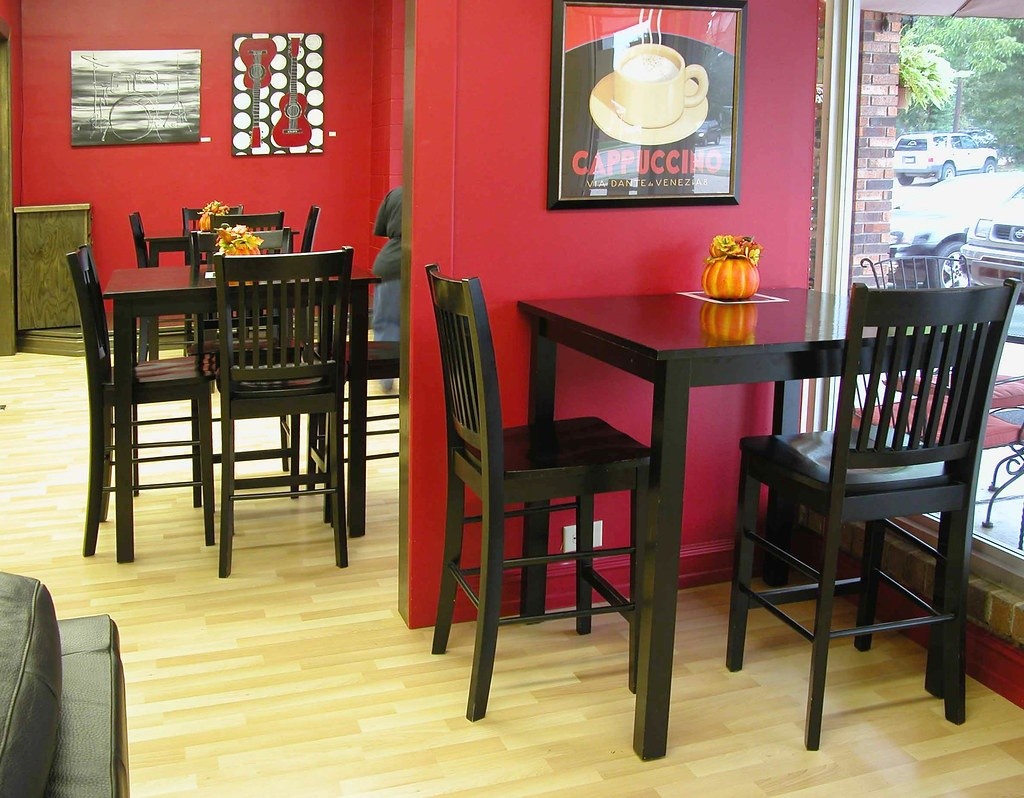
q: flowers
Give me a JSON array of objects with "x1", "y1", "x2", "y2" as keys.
[
  {"x1": 211, "y1": 225, "x2": 265, "y2": 287},
  {"x1": 700, "y1": 234, "x2": 765, "y2": 300},
  {"x1": 196, "y1": 198, "x2": 231, "y2": 234}
]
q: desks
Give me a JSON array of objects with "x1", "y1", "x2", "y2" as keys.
[
  {"x1": 516, "y1": 287, "x2": 978, "y2": 763},
  {"x1": 100, "y1": 253, "x2": 383, "y2": 564}
]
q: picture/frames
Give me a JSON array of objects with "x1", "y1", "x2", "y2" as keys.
[{"x1": 546, "y1": 0, "x2": 747, "y2": 211}]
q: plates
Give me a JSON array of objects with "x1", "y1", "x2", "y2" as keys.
[{"x1": 590, "y1": 72, "x2": 708, "y2": 145}]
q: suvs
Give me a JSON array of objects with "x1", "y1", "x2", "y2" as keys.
[{"x1": 890, "y1": 132, "x2": 999, "y2": 185}]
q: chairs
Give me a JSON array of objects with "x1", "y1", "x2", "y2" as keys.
[
  {"x1": 425, "y1": 263, "x2": 652, "y2": 722},
  {"x1": 726, "y1": 255, "x2": 1024, "y2": 750},
  {"x1": 64, "y1": 206, "x2": 401, "y2": 580}
]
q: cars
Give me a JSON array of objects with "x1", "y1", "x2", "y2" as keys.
[
  {"x1": 888, "y1": 173, "x2": 1024, "y2": 290},
  {"x1": 960, "y1": 181, "x2": 1024, "y2": 343}
]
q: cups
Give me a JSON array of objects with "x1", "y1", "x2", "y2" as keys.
[{"x1": 613, "y1": 43, "x2": 708, "y2": 129}]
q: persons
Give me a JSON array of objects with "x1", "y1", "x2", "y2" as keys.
[{"x1": 369, "y1": 186, "x2": 401, "y2": 393}]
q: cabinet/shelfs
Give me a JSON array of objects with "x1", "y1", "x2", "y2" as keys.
[{"x1": 12, "y1": 202, "x2": 93, "y2": 331}]
